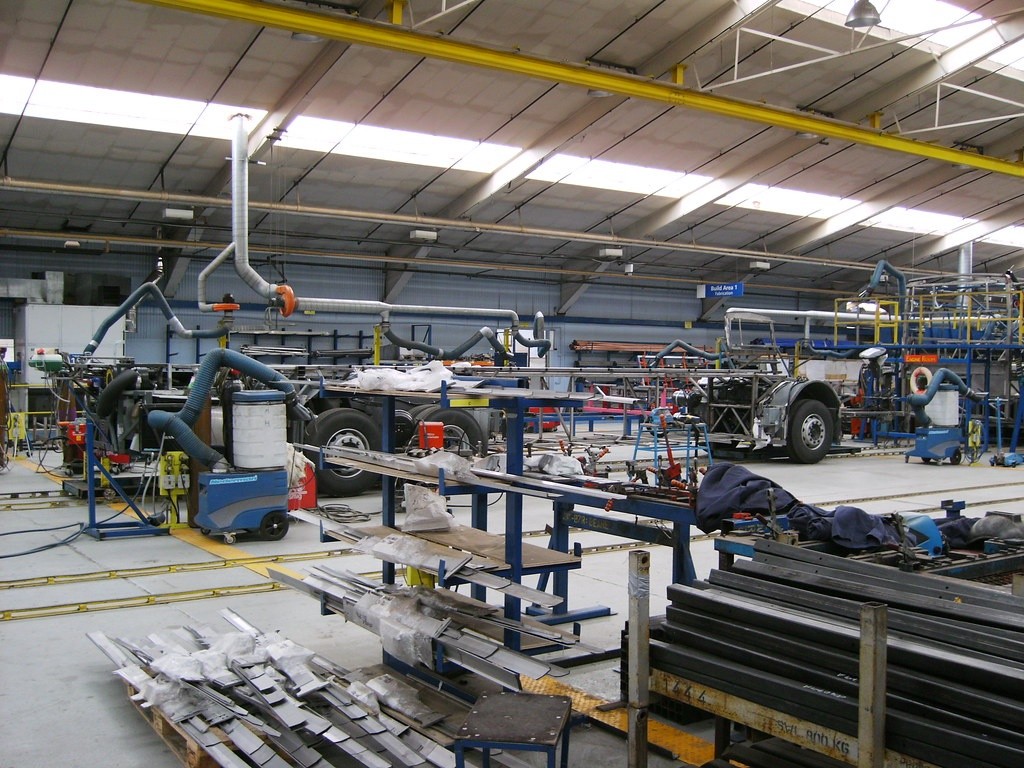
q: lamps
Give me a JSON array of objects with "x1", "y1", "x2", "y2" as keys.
[
  {"x1": 796, "y1": 132, "x2": 818, "y2": 139},
  {"x1": 291, "y1": 32, "x2": 326, "y2": 42},
  {"x1": 845, "y1": 0, "x2": 881, "y2": 27}
]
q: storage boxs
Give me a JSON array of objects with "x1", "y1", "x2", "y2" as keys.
[
  {"x1": 420, "y1": 421, "x2": 444, "y2": 448},
  {"x1": 925, "y1": 385, "x2": 960, "y2": 424},
  {"x1": 232, "y1": 390, "x2": 287, "y2": 468}
]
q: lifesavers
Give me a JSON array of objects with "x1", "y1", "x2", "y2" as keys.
[{"x1": 909, "y1": 366, "x2": 933, "y2": 395}]
